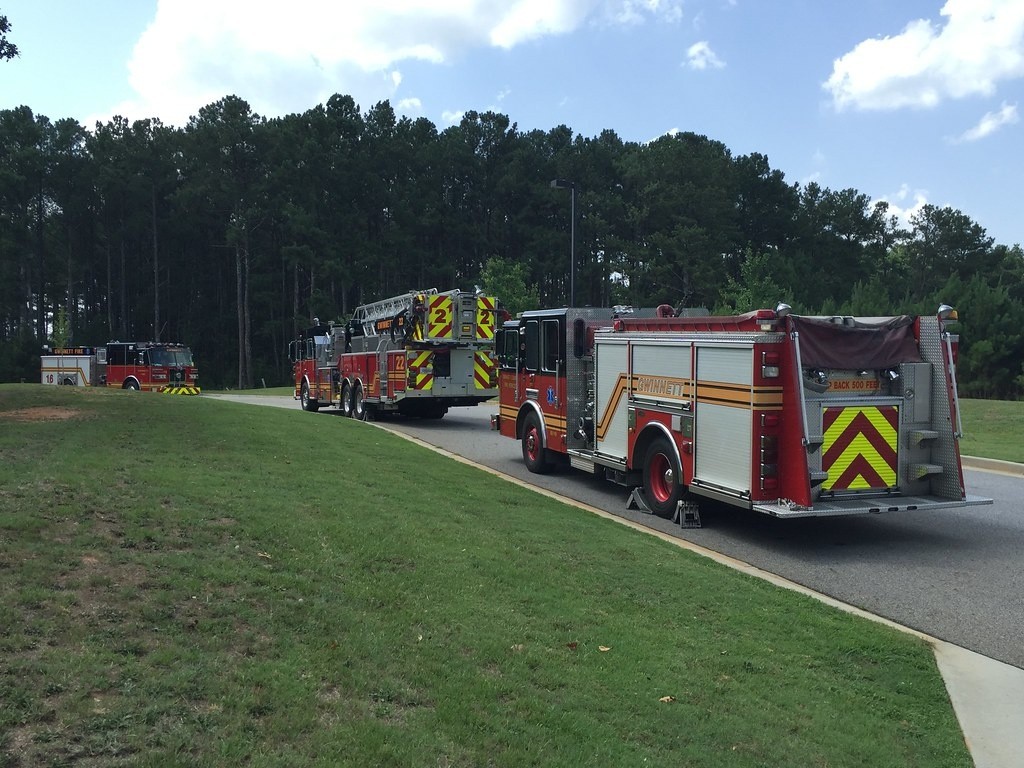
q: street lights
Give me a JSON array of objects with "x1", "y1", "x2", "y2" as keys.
[{"x1": 550, "y1": 179, "x2": 574, "y2": 308}]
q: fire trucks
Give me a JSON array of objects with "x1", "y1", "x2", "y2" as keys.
[
  {"x1": 494, "y1": 301, "x2": 996, "y2": 522},
  {"x1": 287, "y1": 288, "x2": 501, "y2": 423},
  {"x1": 39, "y1": 339, "x2": 201, "y2": 396}
]
